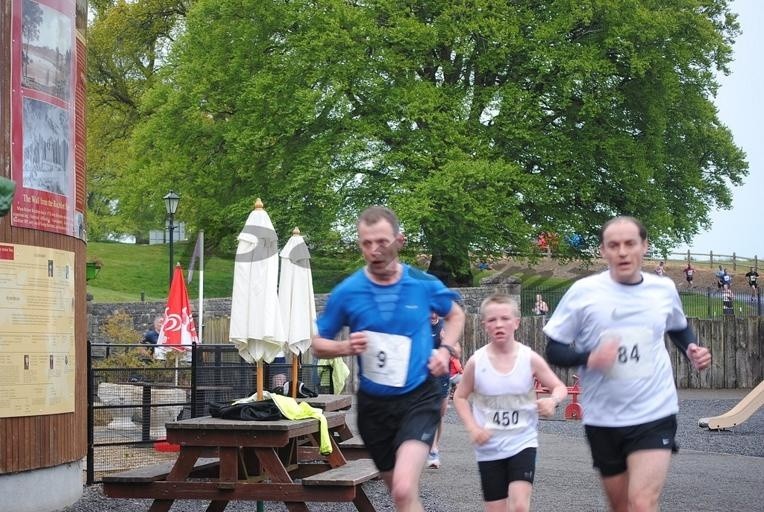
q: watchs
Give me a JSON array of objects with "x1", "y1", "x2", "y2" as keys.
[{"x1": 437, "y1": 342, "x2": 461, "y2": 363}]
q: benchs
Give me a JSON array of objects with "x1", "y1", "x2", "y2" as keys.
[
  {"x1": 306, "y1": 456, "x2": 390, "y2": 512},
  {"x1": 101, "y1": 453, "x2": 225, "y2": 511}
]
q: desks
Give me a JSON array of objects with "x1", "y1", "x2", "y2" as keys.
[
  {"x1": 216, "y1": 388, "x2": 351, "y2": 447},
  {"x1": 160, "y1": 402, "x2": 348, "y2": 512}
]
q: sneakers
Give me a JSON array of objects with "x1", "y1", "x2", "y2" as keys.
[{"x1": 427, "y1": 451, "x2": 440, "y2": 468}]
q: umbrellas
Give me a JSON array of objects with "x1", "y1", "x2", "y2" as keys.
[
  {"x1": 154, "y1": 264, "x2": 200, "y2": 389},
  {"x1": 229, "y1": 196, "x2": 288, "y2": 405},
  {"x1": 278, "y1": 225, "x2": 317, "y2": 405}
]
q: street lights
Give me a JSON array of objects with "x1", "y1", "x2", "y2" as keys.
[{"x1": 163, "y1": 190, "x2": 181, "y2": 286}]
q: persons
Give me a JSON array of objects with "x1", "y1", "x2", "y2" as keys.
[
  {"x1": 427, "y1": 310, "x2": 464, "y2": 474},
  {"x1": 532, "y1": 293, "x2": 551, "y2": 316},
  {"x1": 452, "y1": 295, "x2": 569, "y2": 512},
  {"x1": 682, "y1": 261, "x2": 697, "y2": 286},
  {"x1": 717, "y1": 266, "x2": 725, "y2": 290},
  {"x1": 140, "y1": 316, "x2": 165, "y2": 353},
  {"x1": 311, "y1": 209, "x2": 465, "y2": 512},
  {"x1": 721, "y1": 285, "x2": 735, "y2": 317},
  {"x1": 655, "y1": 261, "x2": 665, "y2": 277},
  {"x1": 541, "y1": 216, "x2": 713, "y2": 512},
  {"x1": 719, "y1": 269, "x2": 732, "y2": 289},
  {"x1": 744, "y1": 268, "x2": 760, "y2": 300}
]
